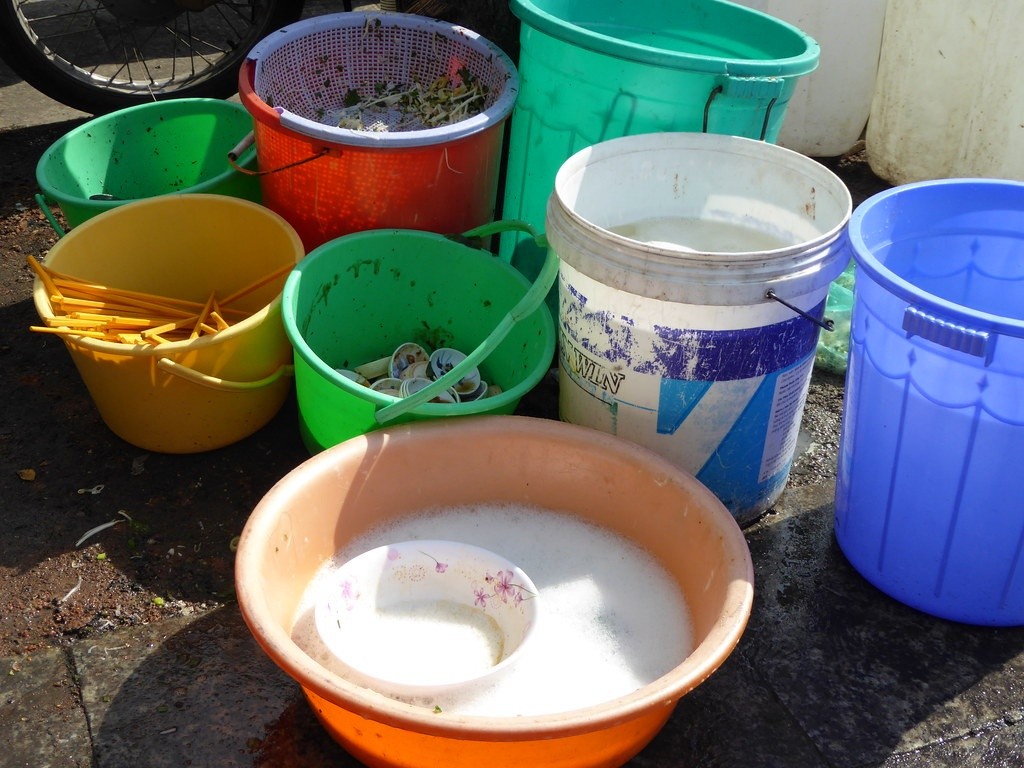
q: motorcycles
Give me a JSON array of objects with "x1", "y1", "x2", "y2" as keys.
[{"x1": 0, "y1": 0, "x2": 519, "y2": 118}]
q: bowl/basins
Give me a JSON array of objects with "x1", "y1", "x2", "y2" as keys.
[
  {"x1": 314, "y1": 539, "x2": 541, "y2": 695},
  {"x1": 235, "y1": 414, "x2": 755, "y2": 768}
]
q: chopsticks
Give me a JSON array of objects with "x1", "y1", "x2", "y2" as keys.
[{"x1": 27, "y1": 252, "x2": 297, "y2": 347}]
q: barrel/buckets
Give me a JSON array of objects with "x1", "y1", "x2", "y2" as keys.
[
  {"x1": 281, "y1": 216, "x2": 559, "y2": 449},
  {"x1": 33, "y1": 196, "x2": 304, "y2": 453},
  {"x1": 546, "y1": 132, "x2": 854, "y2": 527},
  {"x1": 35, "y1": 97, "x2": 262, "y2": 239},
  {"x1": 834, "y1": 178, "x2": 1024, "y2": 626},
  {"x1": 226, "y1": 11, "x2": 519, "y2": 250},
  {"x1": 499, "y1": 1, "x2": 821, "y2": 334}
]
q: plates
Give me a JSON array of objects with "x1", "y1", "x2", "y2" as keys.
[{"x1": 334, "y1": 340, "x2": 502, "y2": 403}]
